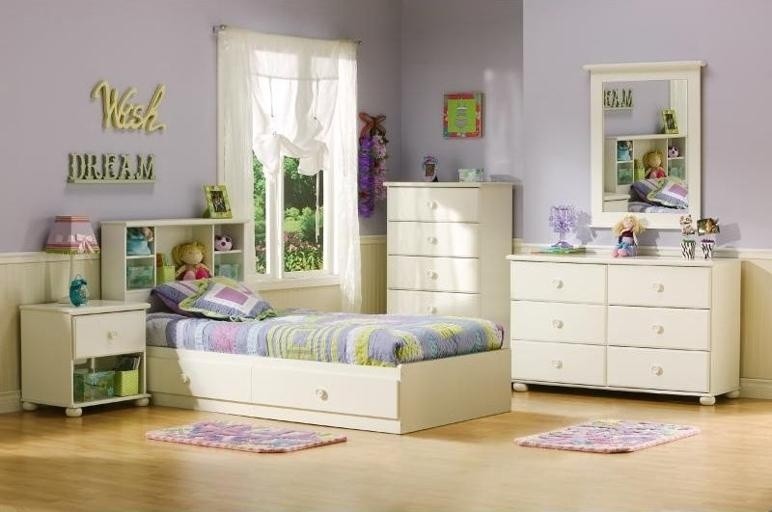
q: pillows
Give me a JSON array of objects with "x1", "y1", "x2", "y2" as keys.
[
  {"x1": 648, "y1": 178, "x2": 688, "y2": 208},
  {"x1": 631, "y1": 177, "x2": 662, "y2": 206},
  {"x1": 149, "y1": 274, "x2": 249, "y2": 319},
  {"x1": 178, "y1": 276, "x2": 277, "y2": 321}
]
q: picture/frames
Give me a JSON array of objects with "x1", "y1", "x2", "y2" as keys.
[
  {"x1": 442, "y1": 92, "x2": 482, "y2": 139},
  {"x1": 201, "y1": 183, "x2": 233, "y2": 219},
  {"x1": 661, "y1": 108, "x2": 679, "y2": 134}
]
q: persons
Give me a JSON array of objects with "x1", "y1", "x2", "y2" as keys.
[
  {"x1": 173, "y1": 240, "x2": 213, "y2": 279},
  {"x1": 644, "y1": 150, "x2": 665, "y2": 178},
  {"x1": 610, "y1": 216, "x2": 640, "y2": 258}
]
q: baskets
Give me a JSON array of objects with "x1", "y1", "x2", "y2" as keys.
[
  {"x1": 124, "y1": 262, "x2": 154, "y2": 290},
  {"x1": 113, "y1": 370, "x2": 138, "y2": 398},
  {"x1": 213, "y1": 263, "x2": 239, "y2": 284},
  {"x1": 158, "y1": 267, "x2": 177, "y2": 284}
]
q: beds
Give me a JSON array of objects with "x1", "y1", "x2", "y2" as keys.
[{"x1": 143, "y1": 307, "x2": 512, "y2": 436}]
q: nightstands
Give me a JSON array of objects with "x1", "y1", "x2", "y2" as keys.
[{"x1": 18, "y1": 297, "x2": 151, "y2": 417}]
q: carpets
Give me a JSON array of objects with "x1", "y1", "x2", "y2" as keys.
[
  {"x1": 145, "y1": 418, "x2": 347, "y2": 453},
  {"x1": 513, "y1": 416, "x2": 701, "y2": 454}
]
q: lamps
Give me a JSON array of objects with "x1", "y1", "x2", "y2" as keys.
[{"x1": 44, "y1": 215, "x2": 99, "y2": 305}]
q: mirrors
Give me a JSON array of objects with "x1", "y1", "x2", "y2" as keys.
[{"x1": 582, "y1": 60, "x2": 706, "y2": 231}]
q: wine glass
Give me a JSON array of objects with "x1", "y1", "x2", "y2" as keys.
[{"x1": 549, "y1": 205, "x2": 576, "y2": 249}]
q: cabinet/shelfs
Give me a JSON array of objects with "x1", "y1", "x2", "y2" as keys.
[
  {"x1": 504, "y1": 250, "x2": 742, "y2": 406},
  {"x1": 383, "y1": 180, "x2": 514, "y2": 349},
  {"x1": 605, "y1": 134, "x2": 687, "y2": 194},
  {"x1": 99, "y1": 217, "x2": 250, "y2": 298}
]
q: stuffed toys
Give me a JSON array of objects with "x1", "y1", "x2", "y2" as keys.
[
  {"x1": 214, "y1": 232, "x2": 234, "y2": 251},
  {"x1": 371, "y1": 127, "x2": 388, "y2": 199},
  {"x1": 669, "y1": 145, "x2": 682, "y2": 159}
]
